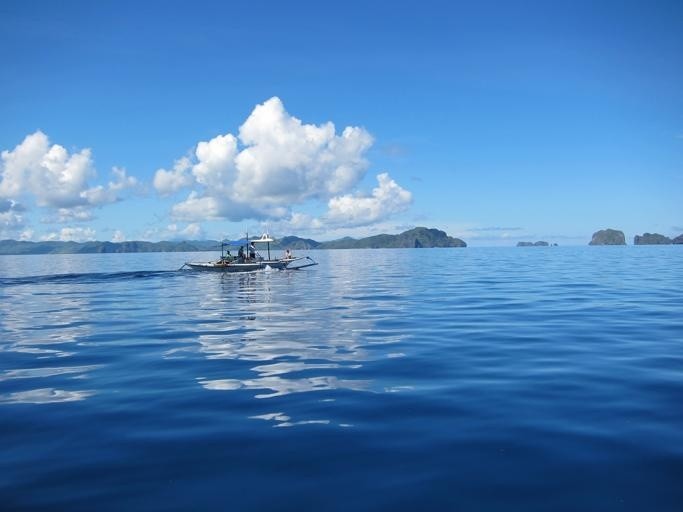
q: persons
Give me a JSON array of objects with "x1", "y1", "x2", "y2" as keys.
[
  {"x1": 240, "y1": 246, "x2": 247, "y2": 262},
  {"x1": 250, "y1": 242, "x2": 257, "y2": 260},
  {"x1": 224, "y1": 250, "x2": 232, "y2": 262},
  {"x1": 286, "y1": 250, "x2": 292, "y2": 260}
]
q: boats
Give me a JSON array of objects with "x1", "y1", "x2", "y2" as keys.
[{"x1": 183, "y1": 230, "x2": 317, "y2": 272}]
